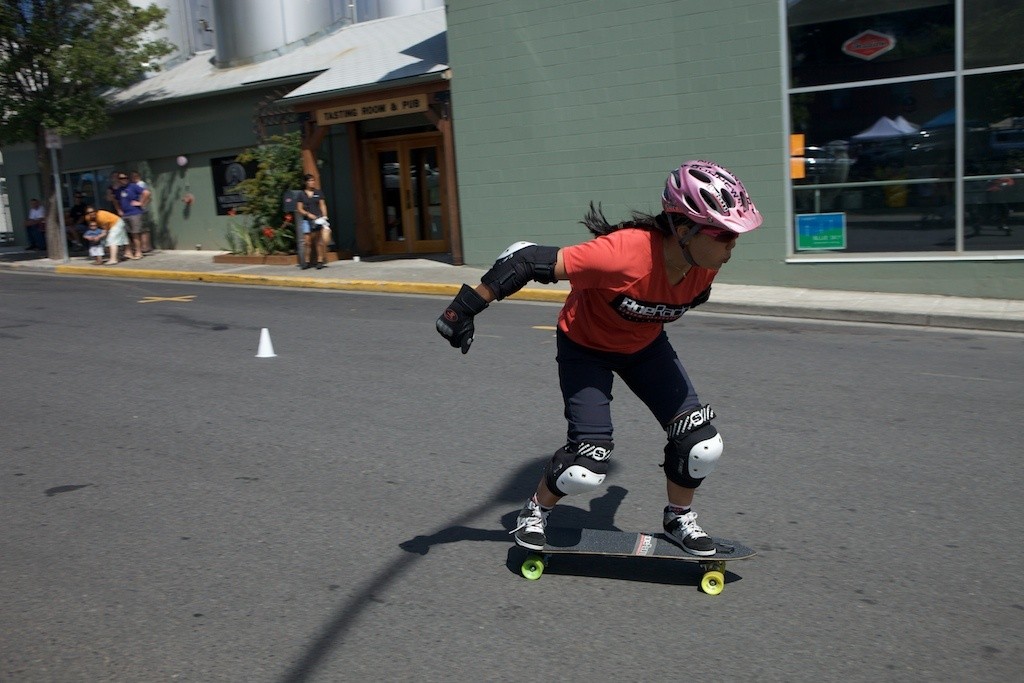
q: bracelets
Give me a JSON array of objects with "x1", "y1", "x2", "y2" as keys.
[{"x1": 303, "y1": 211, "x2": 308, "y2": 217}]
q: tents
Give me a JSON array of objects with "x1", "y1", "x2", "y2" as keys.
[{"x1": 852, "y1": 109, "x2": 956, "y2": 139}]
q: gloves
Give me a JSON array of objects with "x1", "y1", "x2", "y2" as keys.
[{"x1": 436, "y1": 283, "x2": 489, "y2": 354}]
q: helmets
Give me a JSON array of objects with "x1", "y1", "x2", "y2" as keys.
[{"x1": 662, "y1": 159, "x2": 763, "y2": 233}]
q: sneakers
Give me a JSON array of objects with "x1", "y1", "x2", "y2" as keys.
[
  {"x1": 509, "y1": 498, "x2": 552, "y2": 551},
  {"x1": 663, "y1": 507, "x2": 716, "y2": 556}
]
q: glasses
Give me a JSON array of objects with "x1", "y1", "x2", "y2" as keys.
[
  {"x1": 698, "y1": 228, "x2": 740, "y2": 242},
  {"x1": 119, "y1": 177, "x2": 128, "y2": 180}
]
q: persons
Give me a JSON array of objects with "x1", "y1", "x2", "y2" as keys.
[
  {"x1": 82, "y1": 207, "x2": 130, "y2": 267},
  {"x1": 22, "y1": 198, "x2": 48, "y2": 252},
  {"x1": 297, "y1": 174, "x2": 331, "y2": 270},
  {"x1": 435, "y1": 159, "x2": 762, "y2": 556},
  {"x1": 69, "y1": 170, "x2": 151, "y2": 259}
]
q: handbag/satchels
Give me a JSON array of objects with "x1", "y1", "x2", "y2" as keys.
[{"x1": 299, "y1": 220, "x2": 311, "y2": 234}]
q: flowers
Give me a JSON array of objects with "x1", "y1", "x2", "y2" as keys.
[{"x1": 227, "y1": 208, "x2": 295, "y2": 256}]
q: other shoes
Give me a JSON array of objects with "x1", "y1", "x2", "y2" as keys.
[
  {"x1": 304, "y1": 263, "x2": 310, "y2": 269},
  {"x1": 316, "y1": 262, "x2": 323, "y2": 269}
]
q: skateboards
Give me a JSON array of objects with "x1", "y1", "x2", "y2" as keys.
[{"x1": 515, "y1": 526, "x2": 759, "y2": 596}]
